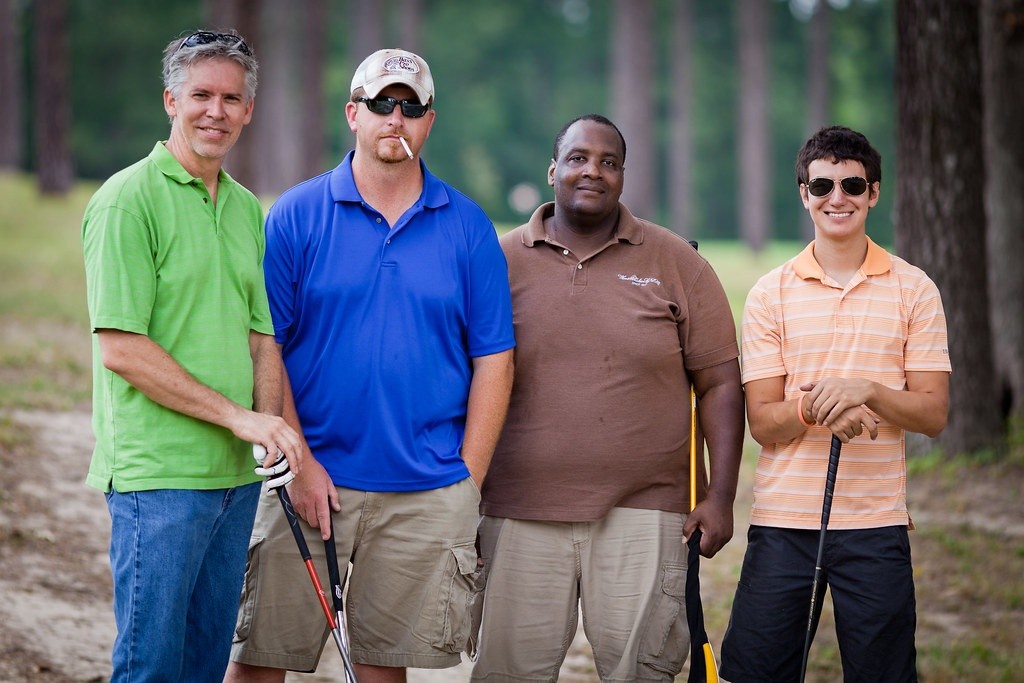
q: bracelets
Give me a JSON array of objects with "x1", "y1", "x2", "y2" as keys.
[{"x1": 798, "y1": 392, "x2": 818, "y2": 429}]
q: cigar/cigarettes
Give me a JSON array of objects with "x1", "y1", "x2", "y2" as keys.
[{"x1": 399, "y1": 135, "x2": 414, "y2": 161}]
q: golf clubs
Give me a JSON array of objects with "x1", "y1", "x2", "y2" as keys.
[
  {"x1": 682, "y1": 236, "x2": 723, "y2": 683},
  {"x1": 269, "y1": 478, "x2": 367, "y2": 683},
  {"x1": 797, "y1": 432, "x2": 844, "y2": 683}
]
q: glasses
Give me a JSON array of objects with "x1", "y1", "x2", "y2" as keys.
[
  {"x1": 356, "y1": 95, "x2": 432, "y2": 119},
  {"x1": 173, "y1": 31, "x2": 253, "y2": 57},
  {"x1": 804, "y1": 176, "x2": 870, "y2": 199}
]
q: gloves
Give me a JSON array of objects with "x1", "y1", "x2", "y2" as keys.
[{"x1": 252, "y1": 443, "x2": 298, "y2": 496}]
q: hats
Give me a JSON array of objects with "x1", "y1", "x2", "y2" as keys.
[{"x1": 350, "y1": 50, "x2": 435, "y2": 106}]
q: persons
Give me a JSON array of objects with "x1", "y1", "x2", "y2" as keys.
[
  {"x1": 454, "y1": 113, "x2": 743, "y2": 681},
  {"x1": 221, "y1": 50, "x2": 517, "y2": 682},
  {"x1": 715, "y1": 131, "x2": 953, "y2": 683},
  {"x1": 82, "y1": 28, "x2": 305, "y2": 683}
]
